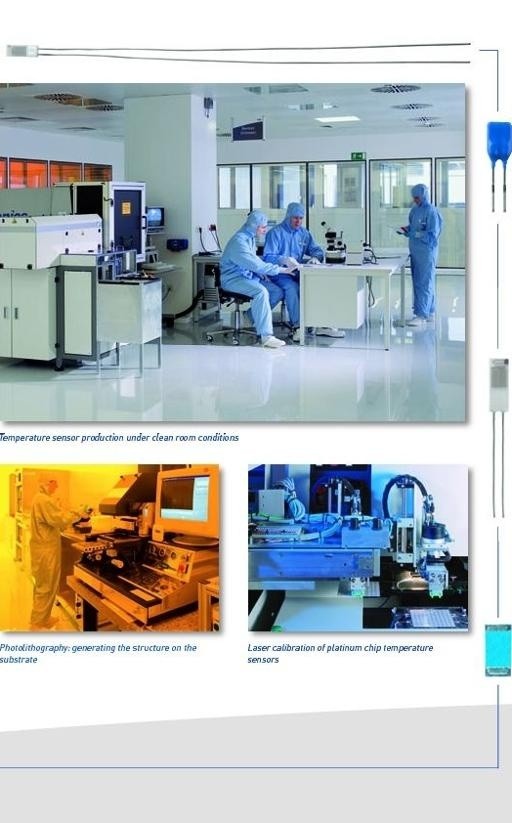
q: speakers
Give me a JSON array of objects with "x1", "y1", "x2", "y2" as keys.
[{"x1": 204, "y1": 97, "x2": 213, "y2": 109}]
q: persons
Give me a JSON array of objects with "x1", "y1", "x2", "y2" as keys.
[
  {"x1": 257, "y1": 199, "x2": 348, "y2": 340},
  {"x1": 22, "y1": 475, "x2": 90, "y2": 632},
  {"x1": 397, "y1": 183, "x2": 443, "y2": 325},
  {"x1": 217, "y1": 208, "x2": 304, "y2": 348}
]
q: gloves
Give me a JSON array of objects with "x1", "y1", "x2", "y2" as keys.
[
  {"x1": 279, "y1": 265, "x2": 298, "y2": 276},
  {"x1": 282, "y1": 257, "x2": 299, "y2": 267},
  {"x1": 307, "y1": 257, "x2": 321, "y2": 265}
]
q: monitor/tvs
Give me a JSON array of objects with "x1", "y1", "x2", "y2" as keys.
[
  {"x1": 145, "y1": 206, "x2": 164, "y2": 226},
  {"x1": 154, "y1": 464, "x2": 220, "y2": 546}
]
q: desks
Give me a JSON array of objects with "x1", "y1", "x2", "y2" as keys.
[{"x1": 296, "y1": 245, "x2": 411, "y2": 351}]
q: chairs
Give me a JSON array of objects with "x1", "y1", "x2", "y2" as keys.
[{"x1": 202, "y1": 263, "x2": 296, "y2": 345}]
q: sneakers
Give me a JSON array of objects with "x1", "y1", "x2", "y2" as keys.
[
  {"x1": 407, "y1": 315, "x2": 425, "y2": 326},
  {"x1": 260, "y1": 334, "x2": 286, "y2": 347},
  {"x1": 293, "y1": 328, "x2": 300, "y2": 341},
  {"x1": 315, "y1": 325, "x2": 346, "y2": 337}
]
